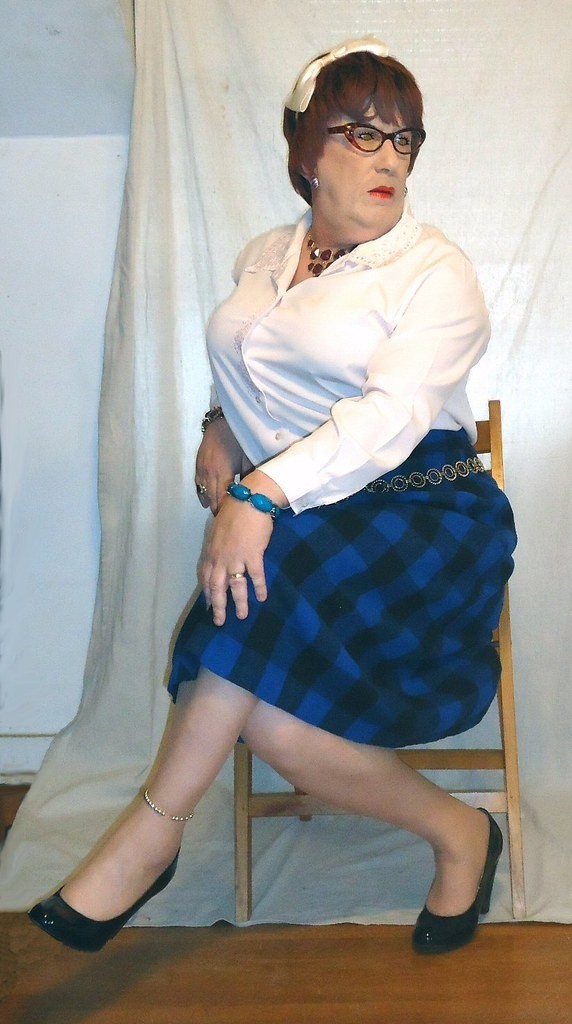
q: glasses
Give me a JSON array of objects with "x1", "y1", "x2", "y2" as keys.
[{"x1": 326, "y1": 122, "x2": 427, "y2": 155}]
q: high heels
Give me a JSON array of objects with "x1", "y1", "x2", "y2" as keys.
[
  {"x1": 28, "y1": 845, "x2": 181, "y2": 953},
  {"x1": 412, "y1": 806, "x2": 503, "y2": 954}
]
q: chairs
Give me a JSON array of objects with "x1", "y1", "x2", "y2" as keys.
[{"x1": 233, "y1": 401, "x2": 526, "y2": 922}]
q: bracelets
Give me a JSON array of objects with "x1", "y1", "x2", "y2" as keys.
[
  {"x1": 225, "y1": 481, "x2": 280, "y2": 518},
  {"x1": 201, "y1": 406, "x2": 224, "y2": 430}
]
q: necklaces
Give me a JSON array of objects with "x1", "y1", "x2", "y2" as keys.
[{"x1": 306, "y1": 231, "x2": 358, "y2": 278}]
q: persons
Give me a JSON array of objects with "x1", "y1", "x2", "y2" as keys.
[{"x1": 27, "y1": 36, "x2": 517, "y2": 955}]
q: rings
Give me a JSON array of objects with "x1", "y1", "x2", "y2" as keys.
[
  {"x1": 229, "y1": 574, "x2": 244, "y2": 579},
  {"x1": 195, "y1": 484, "x2": 207, "y2": 494}
]
